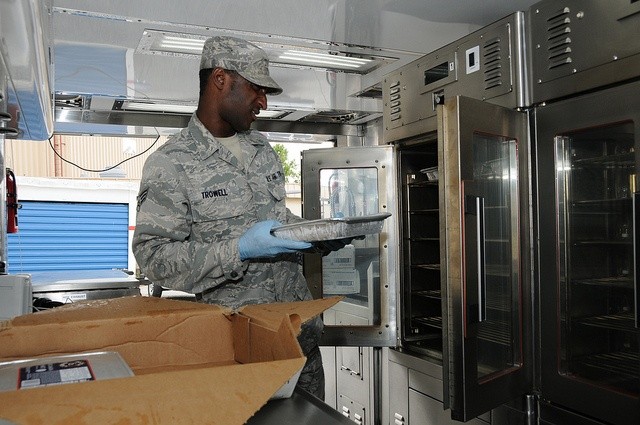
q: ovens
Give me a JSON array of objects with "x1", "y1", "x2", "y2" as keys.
[
  {"x1": 300, "y1": 11, "x2": 528, "y2": 422},
  {"x1": 528, "y1": 0, "x2": 639, "y2": 424}
]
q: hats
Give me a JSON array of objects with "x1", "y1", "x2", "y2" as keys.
[{"x1": 199, "y1": 35, "x2": 283, "y2": 96}]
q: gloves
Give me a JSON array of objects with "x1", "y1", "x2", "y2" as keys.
[{"x1": 237, "y1": 219, "x2": 312, "y2": 261}]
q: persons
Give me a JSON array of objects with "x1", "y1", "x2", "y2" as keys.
[{"x1": 133, "y1": 36, "x2": 366, "y2": 401}]
